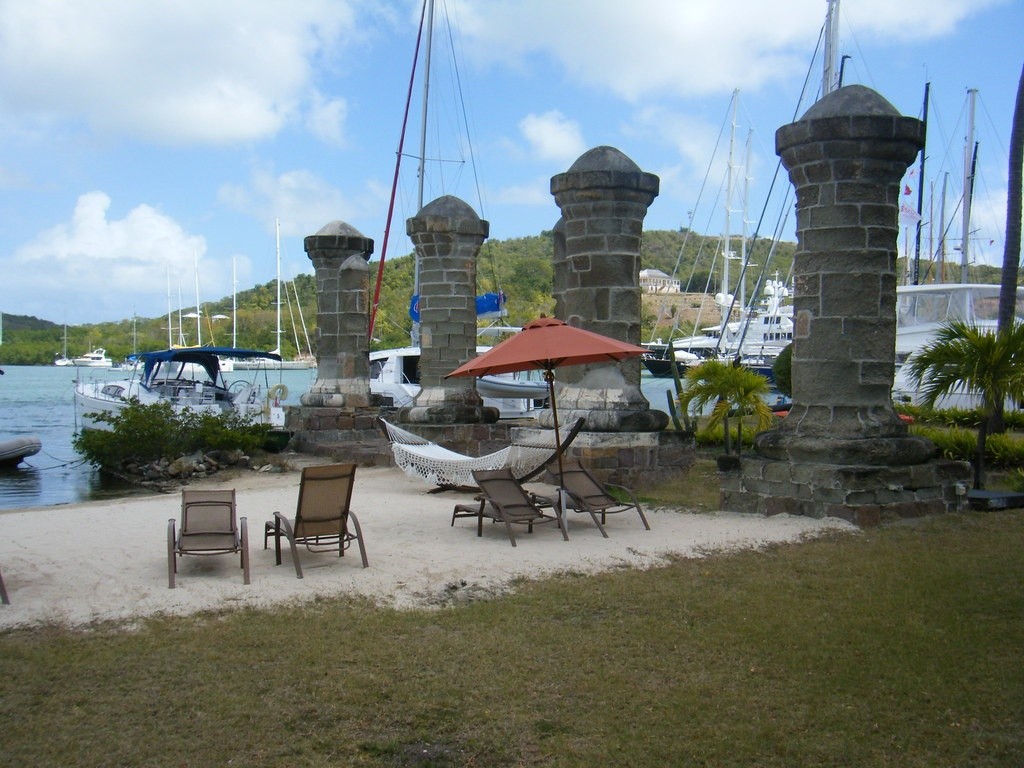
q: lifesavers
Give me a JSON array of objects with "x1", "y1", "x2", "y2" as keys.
[{"x1": 268, "y1": 384, "x2": 289, "y2": 403}]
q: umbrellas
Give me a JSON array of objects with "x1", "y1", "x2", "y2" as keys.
[{"x1": 443, "y1": 313, "x2": 657, "y2": 528}]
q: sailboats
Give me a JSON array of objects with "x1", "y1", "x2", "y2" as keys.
[
  {"x1": 366, "y1": 0, "x2": 551, "y2": 418},
  {"x1": 108, "y1": 219, "x2": 315, "y2": 372},
  {"x1": 640, "y1": 0, "x2": 1024, "y2": 413}
]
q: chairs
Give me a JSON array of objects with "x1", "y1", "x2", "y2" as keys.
[
  {"x1": 265, "y1": 462, "x2": 368, "y2": 579},
  {"x1": 168, "y1": 488, "x2": 250, "y2": 588},
  {"x1": 451, "y1": 467, "x2": 569, "y2": 547},
  {"x1": 535, "y1": 459, "x2": 651, "y2": 538}
]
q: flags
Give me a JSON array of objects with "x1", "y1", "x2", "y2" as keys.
[
  {"x1": 908, "y1": 166, "x2": 920, "y2": 179},
  {"x1": 899, "y1": 204, "x2": 922, "y2": 227},
  {"x1": 905, "y1": 186, "x2": 913, "y2": 196}
]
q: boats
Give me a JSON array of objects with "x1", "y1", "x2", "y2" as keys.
[
  {"x1": 73, "y1": 347, "x2": 289, "y2": 435},
  {"x1": 54, "y1": 348, "x2": 113, "y2": 368}
]
